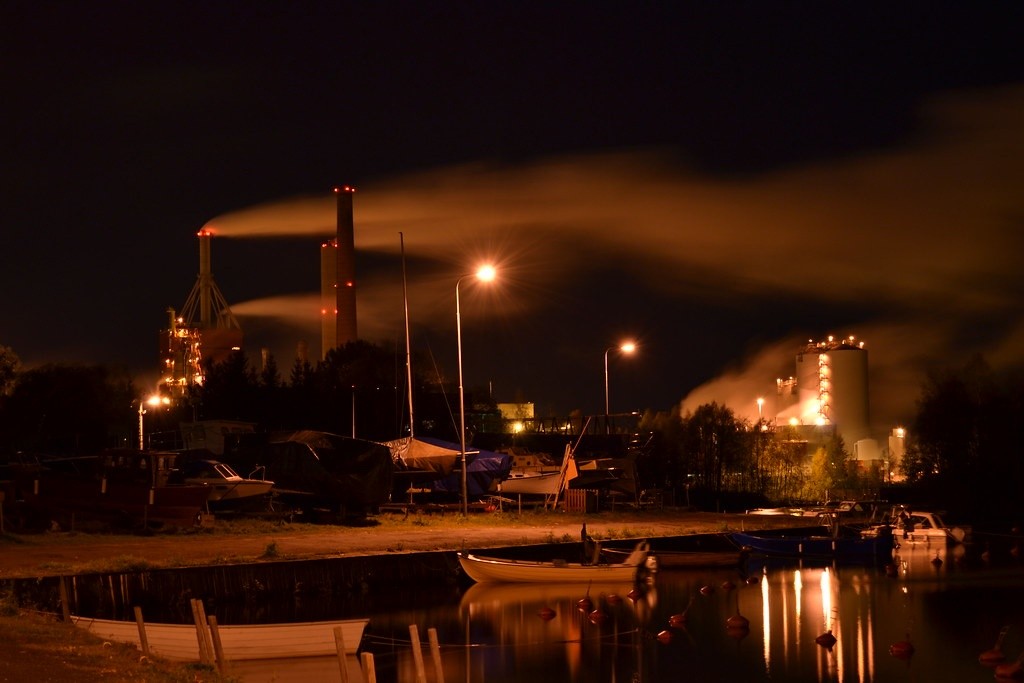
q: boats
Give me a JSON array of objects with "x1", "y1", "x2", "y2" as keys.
[
  {"x1": 457, "y1": 511, "x2": 951, "y2": 581},
  {"x1": 233, "y1": 430, "x2": 637, "y2": 506},
  {"x1": 20, "y1": 609, "x2": 370, "y2": 663},
  {"x1": 47, "y1": 459, "x2": 274, "y2": 529}
]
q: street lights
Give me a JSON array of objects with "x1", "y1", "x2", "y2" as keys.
[
  {"x1": 604, "y1": 342, "x2": 635, "y2": 434},
  {"x1": 138, "y1": 396, "x2": 160, "y2": 451},
  {"x1": 456, "y1": 262, "x2": 498, "y2": 517}
]
